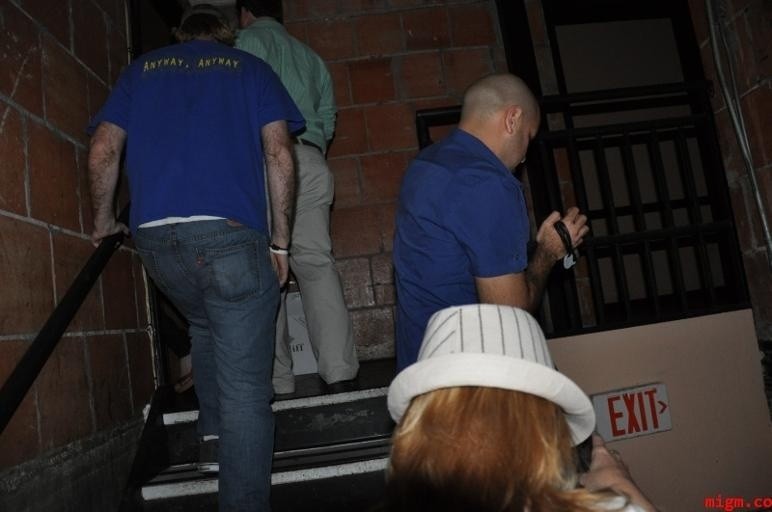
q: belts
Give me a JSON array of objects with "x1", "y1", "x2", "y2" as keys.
[{"x1": 292, "y1": 138, "x2": 323, "y2": 152}]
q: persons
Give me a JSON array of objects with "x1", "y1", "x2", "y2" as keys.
[
  {"x1": 84, "y1": 2, "x2": 360, "y2": 512},
  {"x1": 389, "y1": 73, "x2": 660, "y2": 510}
]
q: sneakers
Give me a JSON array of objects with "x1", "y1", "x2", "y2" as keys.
[
  {"x1": 174, "y1": 368, "x2": 193, "y2": 394},
  {"x1": 199, "y1": 436, "x2": 219, "y2": 473}
]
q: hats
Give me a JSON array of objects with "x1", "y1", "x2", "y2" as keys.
[
  {"x1": 387, "y1": 303, "x2": 596, "y2": 447},
  {"x1": 178, "y1": 3, "x2": 226, "y2": 31}
]
